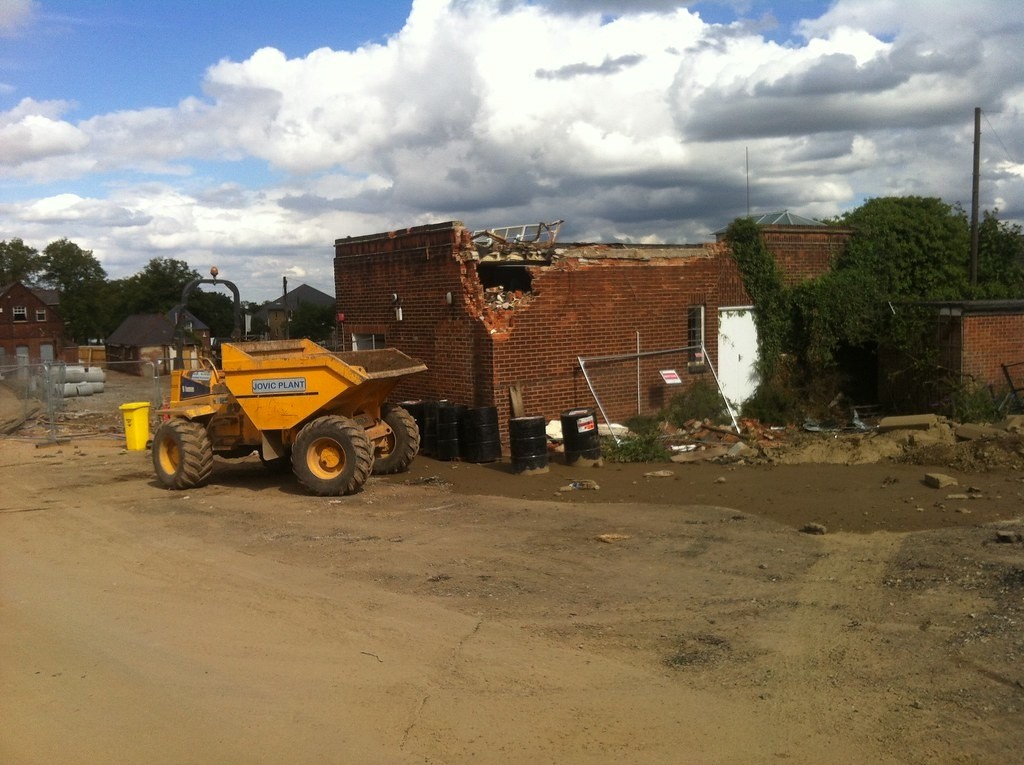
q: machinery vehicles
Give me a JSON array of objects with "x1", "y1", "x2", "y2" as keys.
[{"x1": 151, "y1": 266, "x2": 428, "y2": 497}]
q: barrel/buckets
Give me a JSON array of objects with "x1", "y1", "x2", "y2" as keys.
[
  {"x1": 508, "y1": 416, "x2": 550, "y2": 476},
  {"x1": 401, "y1": 399, "x2": 503, "y2": 462},
  {"x1": 559, "y1": 407, "x2": 604, "y2": 468}
]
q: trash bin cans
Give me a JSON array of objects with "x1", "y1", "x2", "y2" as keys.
[{"x1": 118, "y1": 401, "x2": 150, "y2": 452}]
